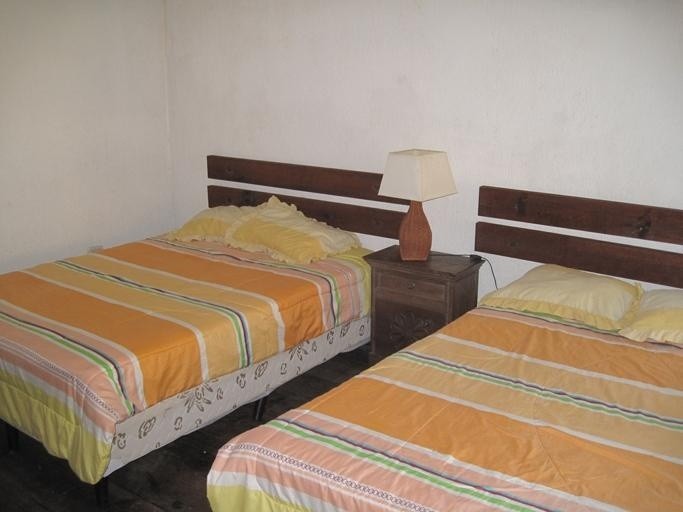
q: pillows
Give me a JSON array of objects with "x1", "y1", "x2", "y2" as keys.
[
  {"x1": 474, "y1": 263, "x2": 683, "y2": 347},
  {"x1": 162, "y1": 194, "x2": 361, "y2": 267}
]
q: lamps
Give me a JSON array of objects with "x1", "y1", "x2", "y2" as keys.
[{"x1": 376, "y1": 148, "x2": 458, "y2": 260}]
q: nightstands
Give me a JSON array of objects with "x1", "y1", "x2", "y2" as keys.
[{"x1": 362, "y1": 245, "x2": 486, "y2": 367}]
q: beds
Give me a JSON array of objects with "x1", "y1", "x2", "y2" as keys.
[
  {"x1": 3, "y1": 154, "x2": 410, "y2": 510},
  {"x1": 207, "y1": 182, "x2": 682, "y2": 510}
]
